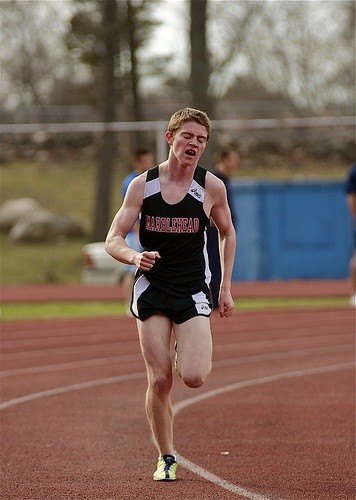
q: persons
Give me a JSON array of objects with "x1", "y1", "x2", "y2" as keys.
[
  {"x1": 105, "y1": 107, "x2": 236, "y2": 481},
  {"x1": 203, "y1": 149, "x2": 238, "y2": 310},
  {"x1": 119, "y1": 146, "x2": 156, "y2": 317},
  {"x1": 343, "y1": 161, "x2": 356, "y2": 309}
]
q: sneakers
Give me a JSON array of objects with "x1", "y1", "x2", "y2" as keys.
[{"x1": 152, "y1": 454, "x2": 178, "y2": 481}]
